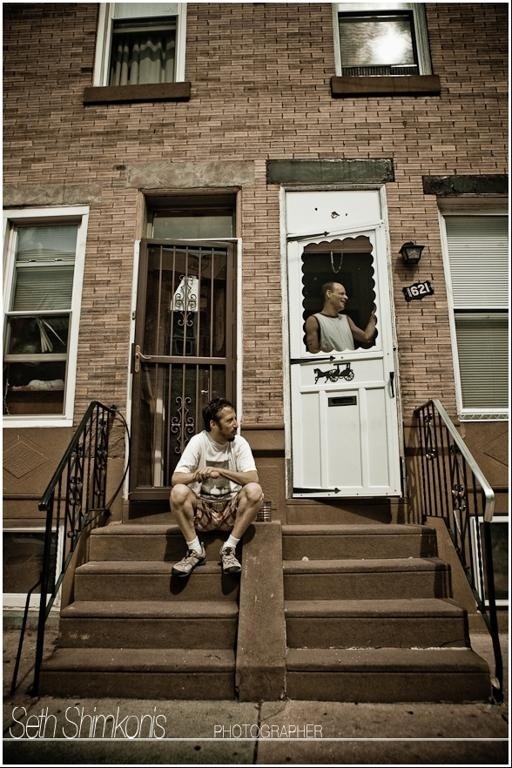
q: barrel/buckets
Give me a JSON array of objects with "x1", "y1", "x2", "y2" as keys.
[{"x1": 257, "y1": 500, "x2": 272, "y2": 521}]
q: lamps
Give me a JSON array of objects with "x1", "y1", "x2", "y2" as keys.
[{"x1": 399, "y1": 241, "x2": 425, "y2": 266}]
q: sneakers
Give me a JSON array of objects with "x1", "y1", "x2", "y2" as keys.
[
  {"x1": 219, "y1": 547, "x2": 241, "y2": 575},
  {"x1": 171, "y1": 541, "x2": 208, "y2": 577}
]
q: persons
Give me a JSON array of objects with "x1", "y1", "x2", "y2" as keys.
[
  {"x1": 305, "y1": 282, "x2": 377, "y2": 353},
  {"x1": 169, "y1": 399, "x2": 264, "y2": 578}
]
